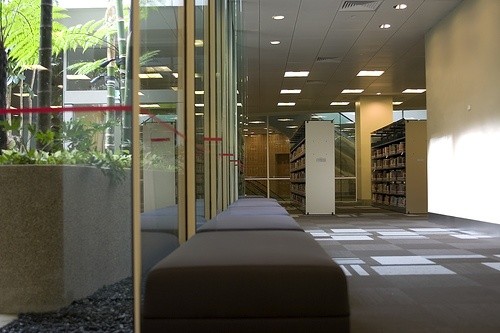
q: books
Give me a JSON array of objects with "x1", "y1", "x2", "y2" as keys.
[
  {"x1": 370, "y1": 138, "x2": 409, "y2": 210},
  {"x1": 289, "y1": 141, "x2": 307, "y2": 213}
]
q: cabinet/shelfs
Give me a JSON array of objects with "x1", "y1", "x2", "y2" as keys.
[
  {"x1": 368, "y1": 119, "x2": 428, "y2": 214},
  {"x1": 288, "y1": 119, "x2": 337, "y2": 216}
]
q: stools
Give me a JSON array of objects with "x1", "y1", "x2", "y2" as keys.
[{"x1": 147, "y1": 196, "x2": 350, "y2": 332}]
